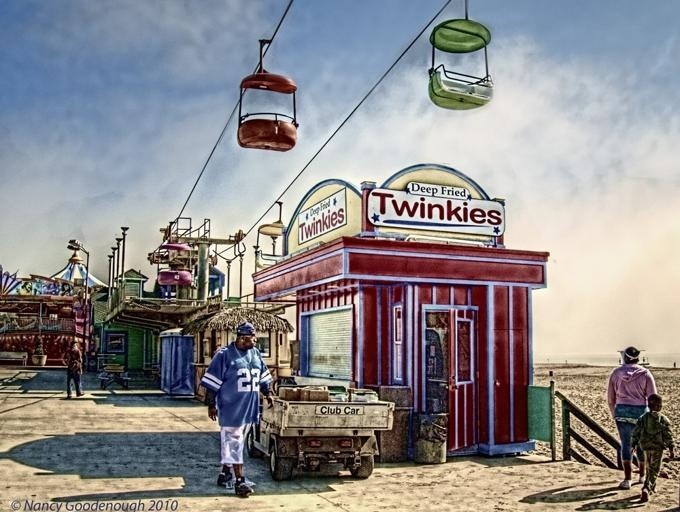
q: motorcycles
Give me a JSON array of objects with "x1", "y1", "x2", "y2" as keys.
[{"x1": 246, "y1": 365, "x2": 397, "y2": 484}]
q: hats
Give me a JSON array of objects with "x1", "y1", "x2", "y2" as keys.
[{"x1": 236, "y1": 321, "x2": 256, "y2": 337}]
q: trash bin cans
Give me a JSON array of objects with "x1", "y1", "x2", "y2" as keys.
[{"x1": 414, "y1": 412, "x2": 449, "y2": 464}]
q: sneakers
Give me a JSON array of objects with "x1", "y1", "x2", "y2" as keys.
[
  {"x1": 216, "y1": 469, "x2": 254, "y2": 497},
  {"x1": 619, "y1": 476, "x2": 656, "y2": 503}
]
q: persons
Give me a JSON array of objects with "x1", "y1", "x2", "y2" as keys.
[
  {"x1": 63, "y1": 342, "x2": 84, "y2": 398},
  {"x1": 202, "y1": 322, "x2": 273, "y2": 496},
  {"x1": 608, "y1": 347, "x2": 675, "y2": 500}
]
